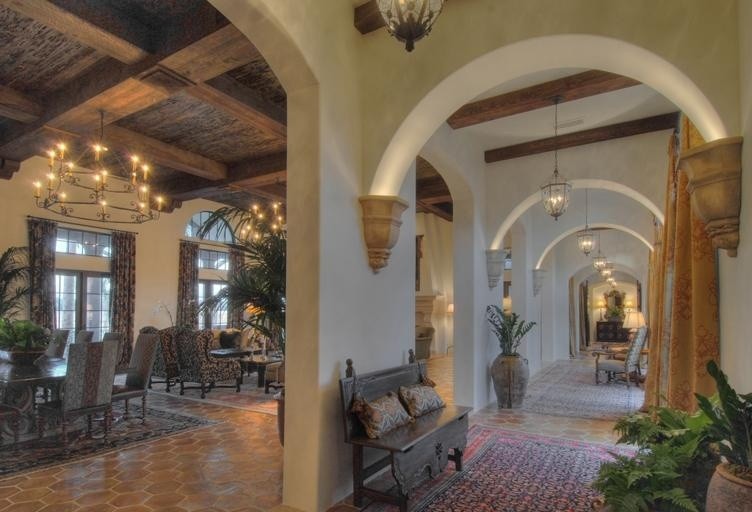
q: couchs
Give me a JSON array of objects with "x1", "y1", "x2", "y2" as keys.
[{"x1": 159, "y1": 323, "x2": 284, "y2": 398}]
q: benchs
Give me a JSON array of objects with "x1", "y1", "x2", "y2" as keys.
[{"x1": 339, "y1": 347, "x2": 474, "y2": 510}]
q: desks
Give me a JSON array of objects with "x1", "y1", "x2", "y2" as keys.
[{"x1": 0, "y1": 355, "x2": 72, "y2": 452}]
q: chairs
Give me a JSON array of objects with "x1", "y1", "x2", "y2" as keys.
[
  {"x1": 44, "y1": 327, "x2": 68, "y2": 358},
  {"x1": 75, "y1": 330, "x2": 93, "y2": 342},
  {"x1": 112, "y1": 321, "x2": 160, "y2": 426},
  {"x1": 0, "y1": 406, "x2": 18, "y2": 455},
  {"x1": 102, "y1": 331, "x2": 122, "y2": 379},
  {"x1": 588, "y1": 321, "x2": 649, "y2": 388},
  {"x1": 36, "y1": 339, "x2": 118, "y2": 459}
]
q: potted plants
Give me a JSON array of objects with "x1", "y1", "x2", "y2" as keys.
[
  {"x1": 197, "y1": 227, "x2": 285, "y2": 447},
  {"x1": 0, "y1": 317, "x2": 48, "y2": 364},
  {"x1": 689, "y1": 355, "x2": 752, "y2": 512},
  {"x1": 485, "y1": 301, "x2": 539, "y2": 408}
]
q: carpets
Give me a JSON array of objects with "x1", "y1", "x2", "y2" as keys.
[
  {"x1": 482, "y1": 366, "x2": 646, "y2": 423},
  {"x1": 146, "y1": 371, "x2": 279, "y2": 415},
  {"x1": 1, "y1": 398, "x2": 223, "y2": 478},
  {"x1": 330, "y1": 421, "x2": 645, "y2": 510}
]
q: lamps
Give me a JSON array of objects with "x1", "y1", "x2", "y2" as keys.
[
  {"x1": 358, "y1": 193, "x2": 410, "y2": 273},
  {"x1": 377, "y1": 1, "x2": 446, "y2": 52},
  {"x1": 33, "y1": 109, "x2": 163, "y2": 223},
  {"x1": 575, "y1": 187, "x2": 596, "y2": 257},
  {"x1": 673, "y1": 136, "x2": 742, "y2": 258},
  {"x1": 540, "y1": 94, "x2": 575, "y2": 222},
  {"x1": 485, "y1": 246, "x2": 507, "y2": 290},
  {"x1": 593, "y1": 229, "x2": 607, "y2": 273},
  {"x1": 446, "y1": 303, "x2": 454, "y2": 317}
]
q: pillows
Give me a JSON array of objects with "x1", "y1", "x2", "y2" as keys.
[
  {"x1": 398, "y1": 382, "x2": 447, "y2": 417},
  {"x1": 354, "y1": 388, "x2": 413, "y2": 440}
]
